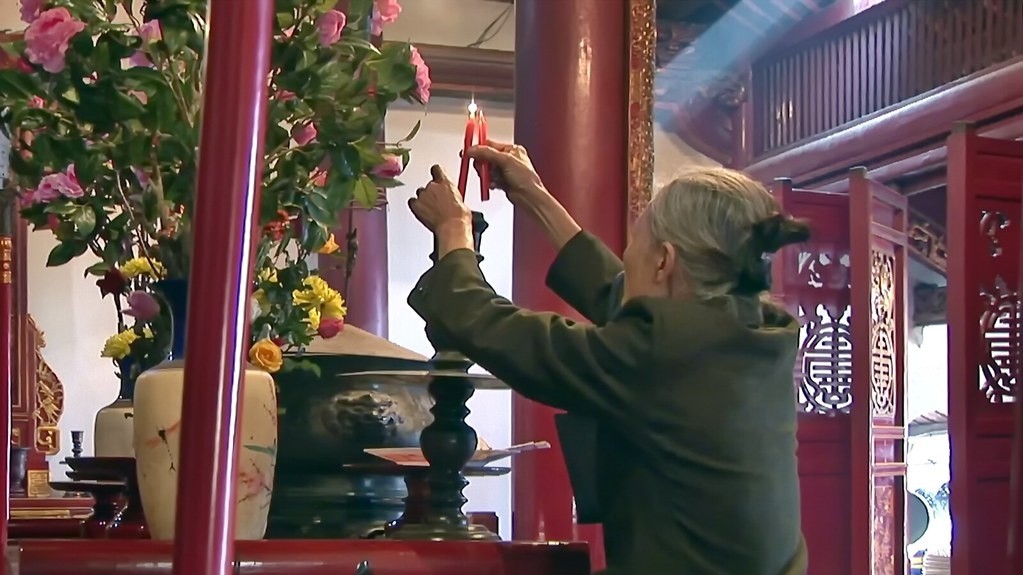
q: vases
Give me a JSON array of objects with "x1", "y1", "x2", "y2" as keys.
[
  {"x1": 93, "y1": 344, "x2": 149, "y2": 457},
  {"x1": 133, "y1": 280, "x2": 278, "y2": 540}
]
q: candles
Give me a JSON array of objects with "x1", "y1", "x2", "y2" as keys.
[
  {"x1": 478, "y1": 110, "x2": 490, "y2": 201},
  {"x1": 458, "y1": 103, "x2": 475, "y2": 203}
]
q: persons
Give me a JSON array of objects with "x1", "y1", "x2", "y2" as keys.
[{"x1": 407, "y1": 140, "x2": 810, "y2": 575}]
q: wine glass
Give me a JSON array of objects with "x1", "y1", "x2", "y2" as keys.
[{"x1": 10, "y1": 447, "x2": 31, "y2": 493}]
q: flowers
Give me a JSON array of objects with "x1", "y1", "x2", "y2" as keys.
[{"x1": 0, "y1": 0, "x2": 430, "y2": 376}]
